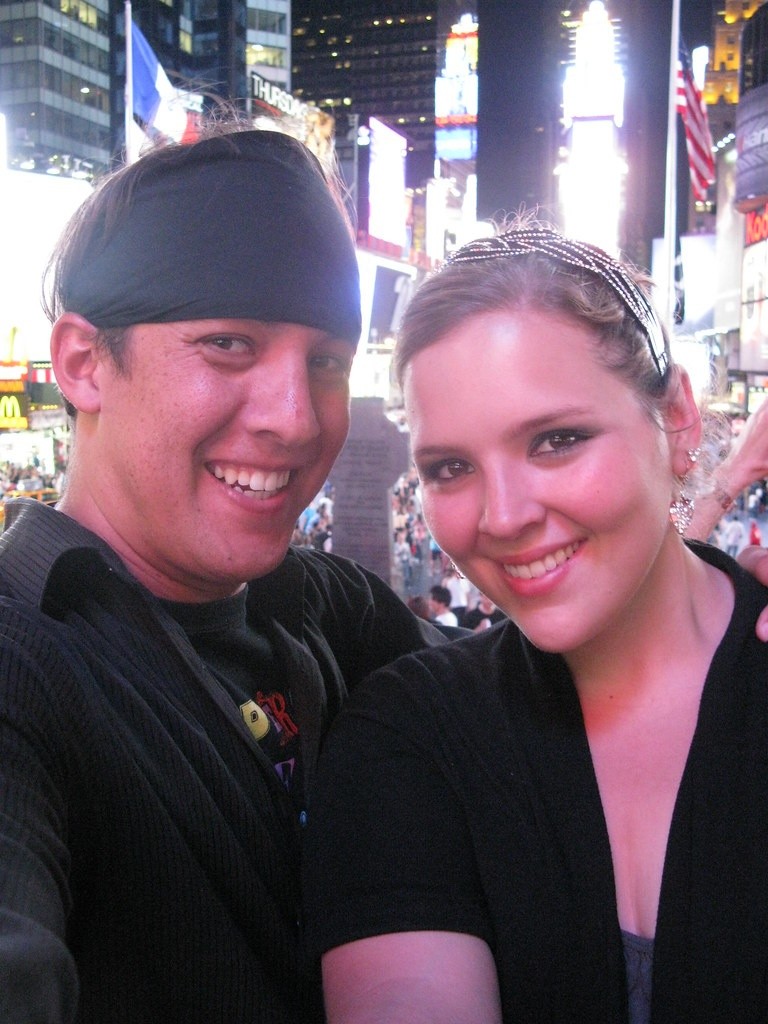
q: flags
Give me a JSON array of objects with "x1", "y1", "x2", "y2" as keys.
[
  {"x1": 676, "y1": 34, "x2": 716, "y2": 202},
  {"x1": 128, "y1": 19, "x2": 201, "y2": 143}
]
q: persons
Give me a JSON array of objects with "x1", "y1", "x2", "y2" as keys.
[
  {"x1": 305, "y1": 227, "x2": 768, "y2": 1024},
  {"x1": 390, "y1": 462, "x2": 508, "y2": 632},
  {"x1": 0, "y1": 132, "x2": 768, "y2": 1023},
  {"x1": 0, "y1": 460, "x2": 50, "y2": 499},
  {"x1": 725, "y1": 516, "x2": 745, "y2": 556},
  {"x1": 748, "y1": 519, "x2": 762, "y2": 546},
  {"x1": 292, "y1": 495, "x2": 332, "y2": 553}
]
